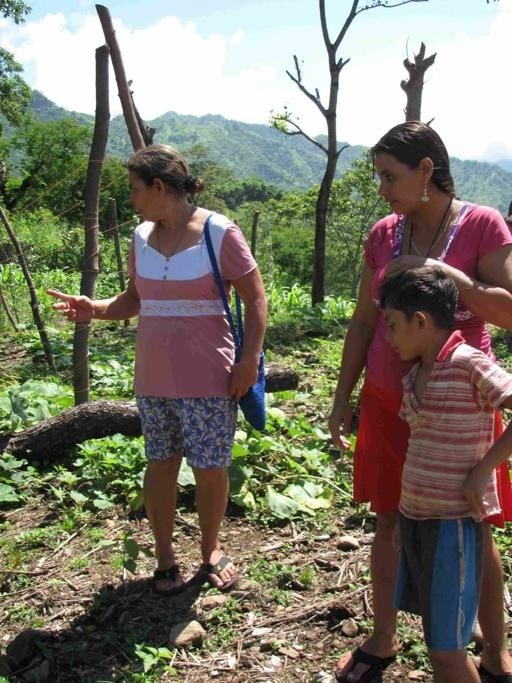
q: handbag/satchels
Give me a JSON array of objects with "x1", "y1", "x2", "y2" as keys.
[{"x1": 238, "y1": 351, "x2": 264, "y2": 430}]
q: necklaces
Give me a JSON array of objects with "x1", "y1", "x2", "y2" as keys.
[
  {"x1": 410, "y1": 198, "x2": 456, "y2": 256},
  {"x1": 157, "y1": 206, "x2": 194, "y2": 258},
  {"x1": 408, "y1": 196, "x2": 453, "y2": 258}
]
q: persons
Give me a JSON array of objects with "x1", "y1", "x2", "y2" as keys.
[
  {"x1": 45, "y1": 145, "x2": 268, "y2": 597},
  {"x1": 328, "y1": 121, "x2": 512, "y2": 683},
  {"x1": 377, "y1": 266, "x2": 512, "y2": 683}
]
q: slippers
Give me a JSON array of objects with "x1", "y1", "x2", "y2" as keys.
[
  {"x1": 335, "y1": 645, "x2": 396, "y2": 683},
  {"x1": 154, "y1": 565, "x2": 185, "y2": 596},
  {"x1": 201, "y1": 557, "x2": 238, "y2": 591}
]
